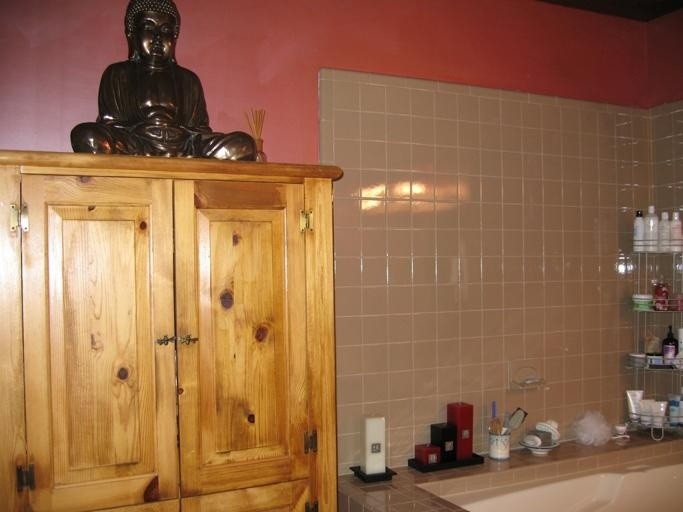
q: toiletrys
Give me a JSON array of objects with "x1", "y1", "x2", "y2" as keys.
[
  {"x1": 627, "y1": 390, "x2": 682, "y2": 426},
  {"x1": 632, "y1": 206, "x2": 683, "y2": 251}
]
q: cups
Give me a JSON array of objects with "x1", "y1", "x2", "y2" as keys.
[{"x1": 489, "y1": 434, "x2": 510, "y2": 459}]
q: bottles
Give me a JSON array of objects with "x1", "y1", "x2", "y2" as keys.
[
  {"x1": 659, "y1": 213, "x2": 672, "y2": 251},
  {"x1": 645, "y1": 204, "x2": 658, "y2": 251},
  {"x1": 662, "y1": 326, "x2": 679, "y2": 365},
  {"x1": 631, "y1": 209, "x2": 645, "y2": 251},
  {"x1": 653, "y1": 273, "x2": 669, "y2": 311},
  {"x1": 672, "y1": 213, "x2": 681, "y2": 248}
]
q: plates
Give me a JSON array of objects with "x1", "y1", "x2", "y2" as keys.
[{"x1": 518, "y1": 440, "x2": 561, "y2": 456}]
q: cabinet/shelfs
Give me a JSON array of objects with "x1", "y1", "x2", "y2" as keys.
[{"x1": 1, "y1": 150, "x2": 344, "y2": 512}]
